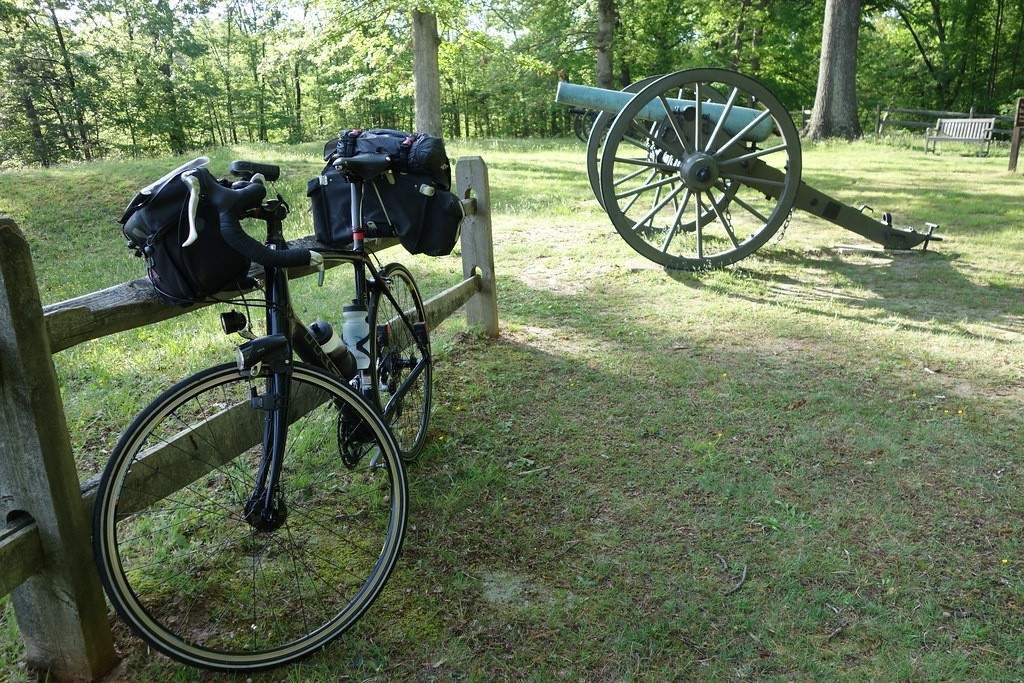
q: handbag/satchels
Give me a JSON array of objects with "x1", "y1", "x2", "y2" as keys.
[
  {"x1": 307, "y1": 169, "x2": 465, "y2": 257},
  {"x1": 124, "y1": 168, "x2": 250, "y2": 307},
  {"x1": 323, "y1": 127, "x2": 452, "y2": 191}
]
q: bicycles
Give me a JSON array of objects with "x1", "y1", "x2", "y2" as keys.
[{"x1": 90, "y1": 159, "x2": 432, "y2": 674}]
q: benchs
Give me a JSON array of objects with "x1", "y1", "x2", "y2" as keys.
[{"x1": 923, "y1": 117, "x2": 996, "y2": 159}]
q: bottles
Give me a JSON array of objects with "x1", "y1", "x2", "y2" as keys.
[
  {"x1": 341, "y1": 298, "x2": 370, "y2": 369},
  {"x1": 307, "y1": 322, "x2": 357, "y2": 379}
]
